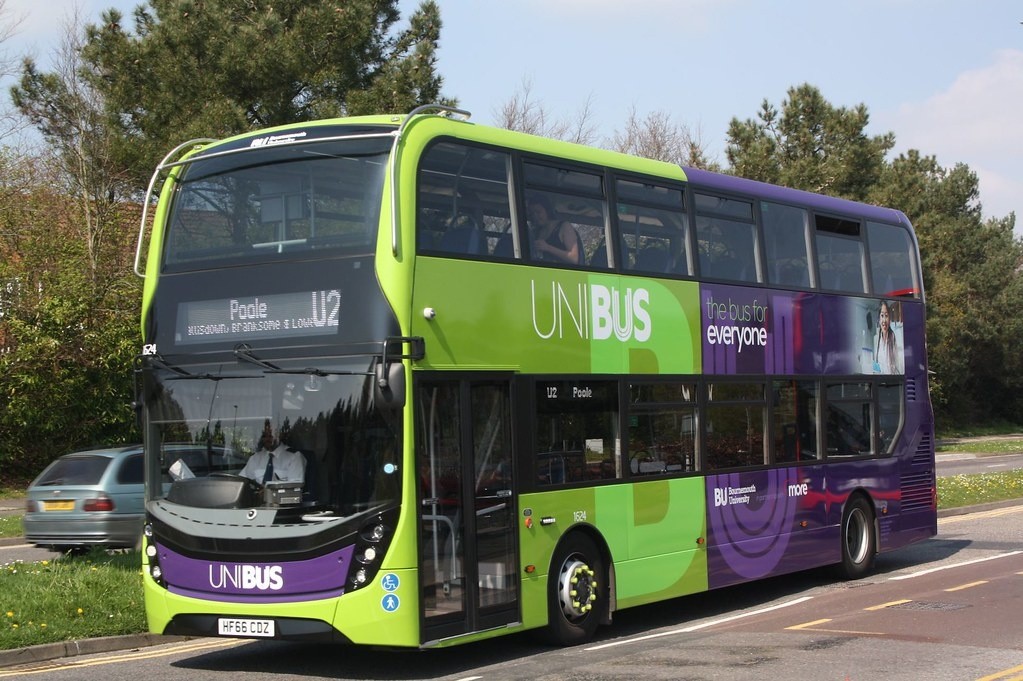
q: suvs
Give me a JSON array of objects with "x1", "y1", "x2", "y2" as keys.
[{"x1": 20, "y1": 440, "x2": 252, "y2": 556}]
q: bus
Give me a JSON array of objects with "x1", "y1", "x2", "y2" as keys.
[{"x1": 143, "y1": 103, "x2": 938, "y2": 650}]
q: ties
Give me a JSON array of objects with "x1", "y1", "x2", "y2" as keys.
[{"x1": 261, "y1": 453, "x2": 274, "y2": 484}]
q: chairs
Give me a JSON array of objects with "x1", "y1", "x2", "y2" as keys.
[
  {"x1": 440, "y1": 215, "x2": 538, "y2": 258},
  {"x1": 295, "y1": 446, "x2": 320, "y2": 495},
  {"x1": 575, "y1": 230, "x2": 897, "y2": 297}
]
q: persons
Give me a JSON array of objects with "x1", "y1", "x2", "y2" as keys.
[
  {"x1": 873, "y1": 301, "x2": 897, "y2": 374},
  {"x1": 421, "y1": 452, "x2": 513, "y2": 593},
  {"x1": 238, "y1": 418, "x2": 307, "y2": 508},
  {"x1": 368, "y1": 445, "x2": 403, "y2": 502},
  {"x1": 522, "y1": 192, "x2": 580, "y2": 266},
  {"x1": 339, "y1": 433, "x2": 376, "y2": 511}
]
